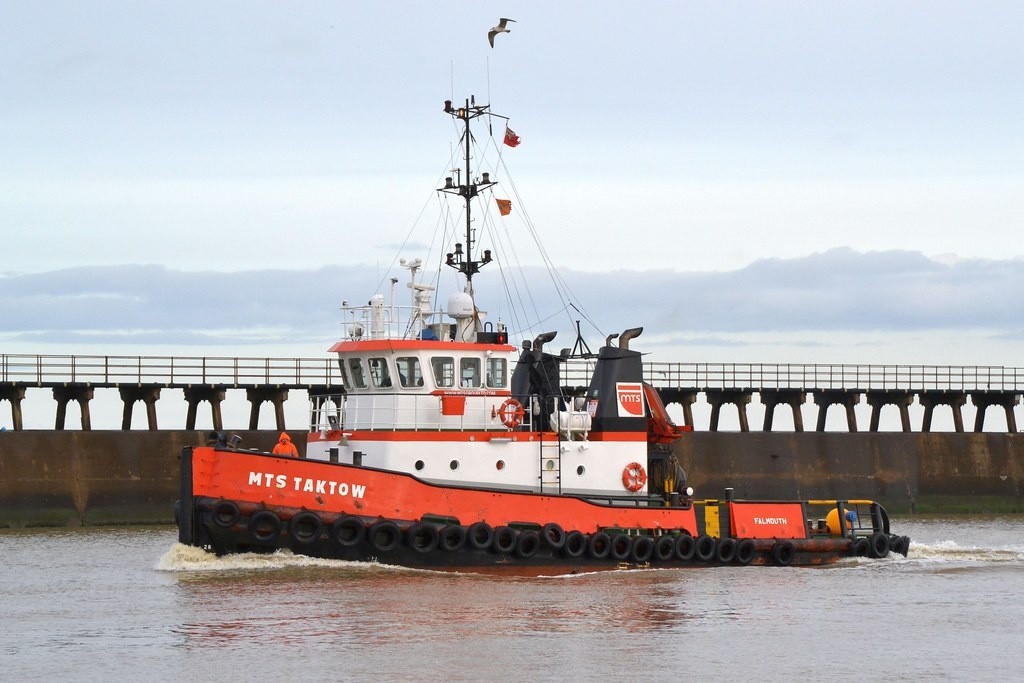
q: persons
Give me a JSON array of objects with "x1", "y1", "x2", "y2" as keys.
[
  {"x1": 661, "y1": 452, "x2": 685, "y2": 493},
  {"x1": 273, "y1": 432, "x2": 298, "y2": 457}
]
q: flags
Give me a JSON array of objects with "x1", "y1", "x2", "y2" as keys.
[
  {"x1": 496, "y1": 199, "x2": 511, "y2": 215},
  {"x1": 504, "y1": 126, "x2": 521, "y2": 147}
]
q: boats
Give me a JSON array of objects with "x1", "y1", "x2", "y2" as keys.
[{"x1": 176, "y1": 57, "x2": 911, "y2": 568}]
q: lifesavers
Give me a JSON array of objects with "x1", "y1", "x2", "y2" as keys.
[
  {"x1": 847, "y1": 533, "x2": 905, "y2": 558},
  {"x1": 467, "y1": 521, "x2": 493, "y2": 550},
  {"x1": 632, "y1": 534, "x2": 795, "y2": 566},
  {"x1": 589, "y1": 531, "x2": 609, "y2": 558},
  {"x1": 287, "y1": 511, "x2": 325, "y2": 545},
  {"x1": 367, "y1": 521, "x2": 402, "y2": 552},
  {"x1": 611, "y1": 535, "x2": 631, "y2": 561},
  {"x1": 517, "y1": 531, "x2": 539, "y2": 559},
  {"x1": 248, "y1": 509, "x2": 280, "y2": 544},
  {"x1": 542, "y1": 522, "x2": 565, "y2": 549},
  {"x1": 211, "y1": 501, "x2": 241, "y2": 527},
  {"x1": 440, "y1": 524, "x2": 466, "y2": 551},
  {"x1": 562, "y1": 530, "x2": 586, "y2": 557},
  {"x1": 492, "y1": 525, "x2": 516, "y2": 553},
  {"x1": 623, "y1": 462, "x2": 646, "y2": 491},
  {"x1": 500, "y1": 400, "x2": 523, "y2": 427},
  {"x1": 331, "y1": 515, "x2": 366, "y2": 547},
  {"x1": 406, "y1": 520, "x2": 439, "y2": 555}
]
